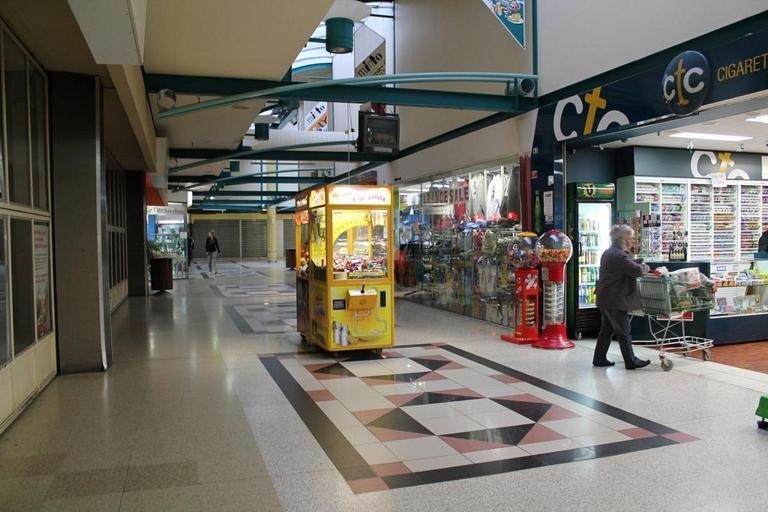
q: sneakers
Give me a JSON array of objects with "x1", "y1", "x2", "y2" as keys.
[{"x1": 209, "y1": 271, "x2": 218, "y2": 275}]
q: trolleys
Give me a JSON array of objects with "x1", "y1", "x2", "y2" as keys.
[{"x1": 631, "y1": 264, "x2": 722, "y2": 373}]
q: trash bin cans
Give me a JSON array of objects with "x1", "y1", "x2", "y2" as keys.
[{"x1": 150, "y1": 258, "x2": 173, "y2": 296}]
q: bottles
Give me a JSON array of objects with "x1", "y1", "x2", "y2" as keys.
[
  {"x1": 579, "y1": 211, "x2": 600, "y2": 306},
  {"x1": 668, "y1": 244, "x2": 688, "y2": 262}
]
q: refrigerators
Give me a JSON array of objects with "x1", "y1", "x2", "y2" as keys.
[{"x1": 566, "y1": 182, "x2": 618, "y2": 340}]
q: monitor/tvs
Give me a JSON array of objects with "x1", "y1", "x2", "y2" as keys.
[{"x1": 359, "y1": 113, "x2": 400, "y2": 153}]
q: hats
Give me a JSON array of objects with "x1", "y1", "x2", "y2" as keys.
[{"x1": 610, "y1": 224, "x2": 631, "y2": 239}]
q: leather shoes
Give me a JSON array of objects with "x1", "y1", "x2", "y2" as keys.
[
  {"x1": 594, "y1": 360, "x2": 616, "y2": 368},
  {"x1": 625, "y1": 358, "x2": 649, "y2": 368}
]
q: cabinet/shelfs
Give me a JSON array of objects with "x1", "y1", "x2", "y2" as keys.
[
  {"x1": 614, "y1": 176, "x2": 768, "y2": 258},
  {"x1": 710, "y1": 262, "x2": 768, "y2": 319}
]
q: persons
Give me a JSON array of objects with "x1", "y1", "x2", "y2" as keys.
[
  {"x1": 592, "y1": 224, "x2": 651, "y2": 371},
  {"x1": 204, "y1": 229, "x2": 220, "y2": 275},
  {"x1": 188, "y1": 233, "x2": 194, "y2": 265},
  {"x1": 401, "y1": 226, "x2": 412, "y2": 243}
]
